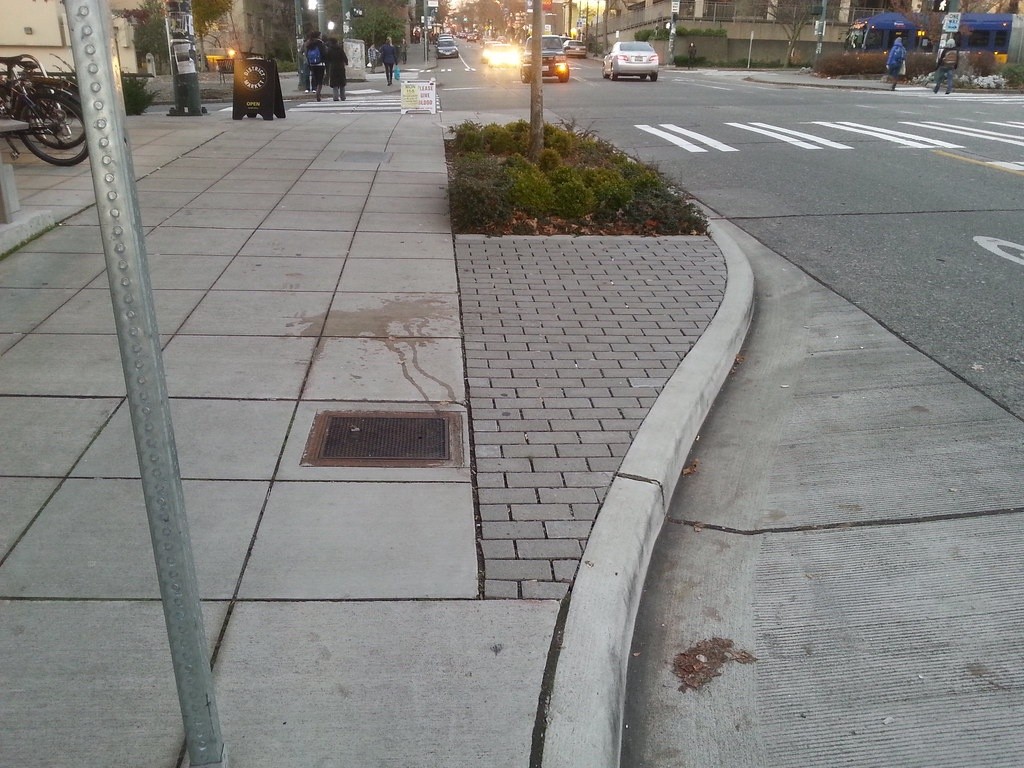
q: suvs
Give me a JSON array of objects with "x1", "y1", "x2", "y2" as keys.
[{"x1": 520, "y1": 34, "x2": 570, "y2": 84}]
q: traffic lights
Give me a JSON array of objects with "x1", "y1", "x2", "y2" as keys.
[
  {"x1": 327, "y1": 20, "x2": 335, "y2": 31},
  {"x1": 302, "y1": 0, "x2": 318, "y2": 15},
  {"x1": 672, "y1": 13, "x2": 679, "y2": 22},
  {"x1": 932, "y1": 0, "x2": 951, "y2": 15},
  {"x1": 662, "y1": 21, "x2": 671, "y2": 29}
]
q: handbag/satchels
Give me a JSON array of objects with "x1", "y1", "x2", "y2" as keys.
[
  {"x1": 898, "y1": 59, "x2": 906, "y2": 75},
  {"x1": 394, "y1": 64, "x2": 400, "y2": 81}
]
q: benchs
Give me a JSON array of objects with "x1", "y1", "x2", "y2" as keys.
[
  {"x1": 0, "y1": 118, "x2": 30, "y2": 227},
  {"x1": 216, "y1": 58, "x2": 234, "y2": 85}
]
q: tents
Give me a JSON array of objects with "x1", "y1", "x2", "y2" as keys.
[{"x1": 847, "y1": 12, "x2": 918, "y2": 50}]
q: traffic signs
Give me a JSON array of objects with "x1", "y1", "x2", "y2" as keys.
[
  {"x1": 944, "y1": 11, "x2": 962, "y2": 33},
  {"x1": 671, "y1": 2, "x2": 680, "y2": 13}
]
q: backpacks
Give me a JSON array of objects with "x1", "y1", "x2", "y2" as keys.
[
  {"x1": 943, "y1": 47, "x2": 958, "y2": 64},
  {"x1": 307, "y1": 41, "x2": 322, "y2": 64}
]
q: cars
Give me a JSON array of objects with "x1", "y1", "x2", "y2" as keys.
[
  {"x1": 554, "y1": 36, "x2": 572, "y2": 45},
  {"x1": 602, "y1": 40, "x2": 659, "y2": 82},
  {"x1": 434, "y1": 31, "x2": 523, "y2": 68},
  {"x1": 563, "y1": 39, "x2": 587, "y2": 58}
]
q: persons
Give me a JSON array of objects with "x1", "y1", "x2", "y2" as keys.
[
  {"x1": 326, "y1": 37, "x2": 349, "y2": 101},
  {"x1": 934, "y1": 39, "x2": 959, "y2": 94},
  {"x1": 920, "y1": 31, "x2": 933, "y2": 53},
  {"x1": 379, "y1": 36, "x2": 398, "y2": 86},
  {"x1": 413, "y1": 27, "x2": 424, "y2": 43},
  {"x1": 844, "y1": 34, "x2": 866, "y2": 55},
  {"x1": 886, "y1": 37, "x2": 907, "y2": 92},
  {"x1": 688, "y1": 42, "x2": 696, "y2": 71},
  {"x1": 367, "y1": 43, "x2": 380, "y2": 73},
  {"x1": 299, "y1": 31, "x2": 328, "y2": 101},
  {"x1": 171, "y1": 20, "x2": 180, "y2": 30},
  {"x1": 428, "y1": 30, "x2": 439, "y2": 44},
  {"x1": 400, "y1": 37, "x2": 407, "y2": 64}
]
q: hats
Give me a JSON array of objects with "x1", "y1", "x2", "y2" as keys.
[{"x1": 894, "y1": 37, "x2": 902, "y2": 43}]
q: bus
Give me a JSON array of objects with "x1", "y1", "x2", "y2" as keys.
[{"x1": 836, "y1": 10, "x2": 1024, "y2": 65}]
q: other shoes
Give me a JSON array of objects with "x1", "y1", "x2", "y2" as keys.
[
  {"x1": 312, "y1": 90, "x2": 317, "y2": 93},
  {"x1": 304, "y1": 90, "x2": 309, "y2": 93},
  {"x1": 341, "y1": 97, "x2": 346, "y2": 101},
  {"x1": 891, "y1": 86, "x2": 895, "y2": 91},
  {"x1": 945, "y1": 90, "x2": 952, "y2": 94},
  {"x1": 317, "y1": 98, "x2": 321, "y2": 102},
  {"x1": 334, "y1": 99, "x2": 339, "y2": 101},
  {"x1": 933, "y1": 88, "x2": 938, "y2": 93}
]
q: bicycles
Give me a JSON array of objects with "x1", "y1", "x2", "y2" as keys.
[{"x1": 0, "y1": 54, "x2": 88, "y2": 167}]
